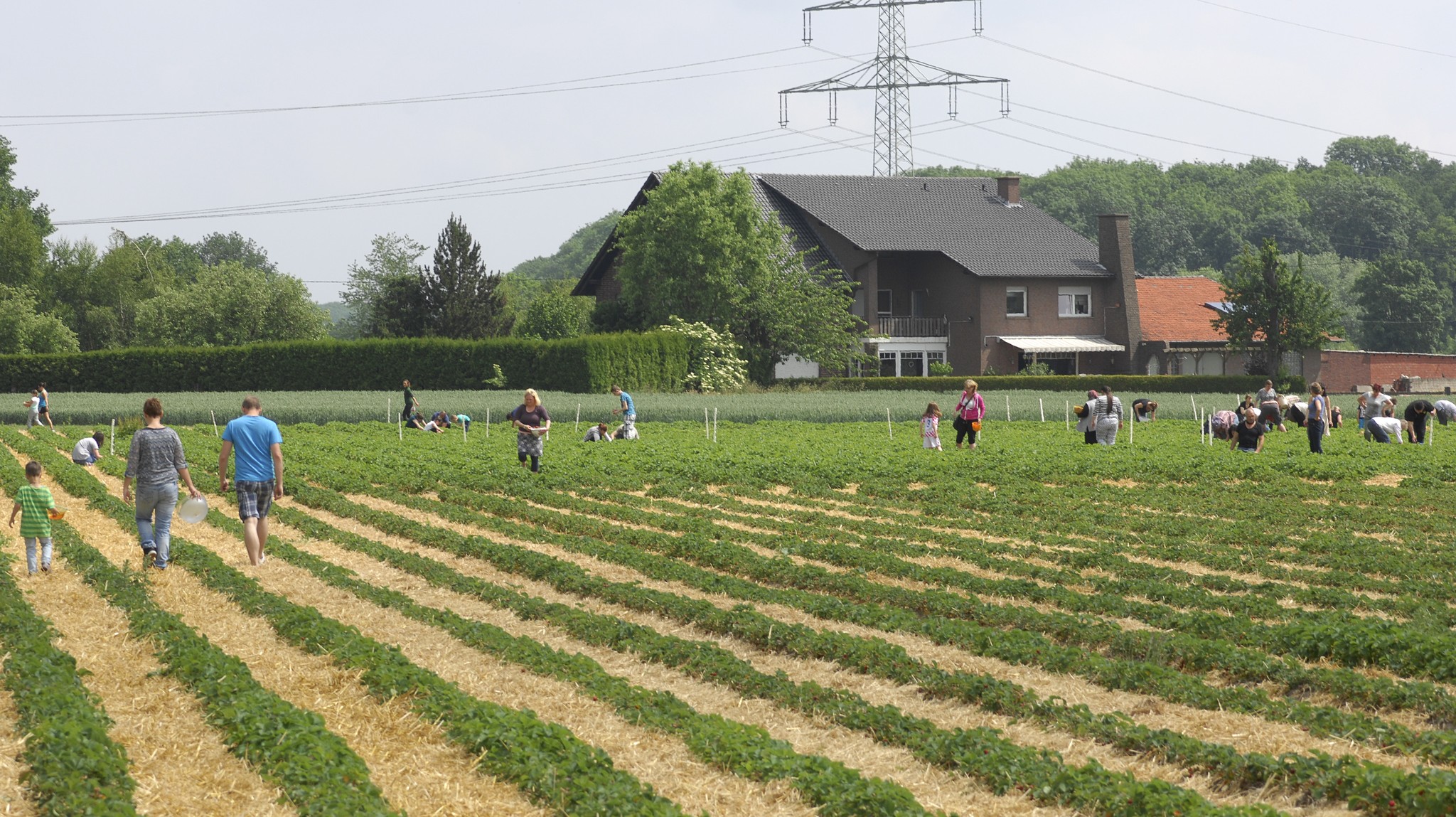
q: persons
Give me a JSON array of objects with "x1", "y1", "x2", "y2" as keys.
[
  {"x1": 1075, "y1": 390, "x2": 1098, "y2": 445},
  {"x1": 1357, "y1": 382, "x2": 1394, "y2": 443},
  {"x1": 505, "y1": 406, "x2": 519, "y2": 429},
  {"x1": 1367, "y1": 416, "x2": 1408, "y2": 444},
  {"x1": 8, "y1": 461, "x2": 61, "y2": 579},
  {"x1": 122, "y1": 397, "x2": 203, "y2": 574},
  {"x1": 218, "y1": 396, "x2": 284, "y2": 567},
  {"x1": 1132, "y1": 399, "x2": 1159, "y2": 423},
  {"x1": 430, "y1": 411, "x2": 451, "y2": 429},
  {"x1": 23, "y1": 389, "x2": 44, "y2": 431},
  {"x1": 952, "y1": 378, "x2": 986, "y2": 451},
  {"x1": 610, "y1": 424, "x2": 640, "y2": 440},
  {"x1": 1404, "y1": 399, "x2": 1436, "y2": 445},
  {"x1": 1434, "y1": 400, "x2": 1456, "y2": 426},
  {"x1": 1307, "y1": 381, "x2": 1325, "y2": 454},
  {"x1": 513, "y1": 388, "x2": 551, "y2": 474},
  {"x1": 405, "y1": 411, "x2": 427, "y2": 432},
  {"x1": 583, "y1": 422, "x2": 612, "y2": 443},
  {"x1": 1091, "y1": 385, "x2": 1124, "y2": 447},
  {"x1": 920, "y1": 402, "x2": 943, "y2": 451},
  {"x1": 1199, "y1": 394, "x2": 1399, "y2": 442},
  {"x1": 424, "y1": 415, "x2": 445, "y2": 434},
  {"x1": 402, "y1": 379, "x2": 420, "y2": 422},
  {"x1": 34, "y1": 381, "x2": 55, "y2": 432},
  {"x1": 1254, "y1": 379, "x2": 1283, "y2": 431},
  {"x1": 1229, "y1": 407, "x2": 1264, "y2": 455},
  {"x1": 610, "y1": 384, "x2": 636, "y2": 440},
  {"x1": 72, "y1": 430, "x2": 105, "y2": 466},
  {"x1": 451, "y1": 413, "x2": 471, "y2": 432},
  {"x1": 1303, "y1": 381, "x2": 1334, "y2": 441}
]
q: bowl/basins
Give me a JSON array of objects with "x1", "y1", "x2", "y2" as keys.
[
  {"x1": 530, "y1": 426, "x2": 546, "y2": 437},
  {"x1": 46, "y1": 504, "x2": 67, "y2": 519},
  {"x1": 177, "y1": 492, "x2": 209, "y2": 523}
]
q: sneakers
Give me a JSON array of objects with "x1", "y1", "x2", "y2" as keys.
[
  {"x1": 41, "y1": 563, "x2": 50, "y2": 576},
  {"x1": 258, "y1": 552, "x2": 265, "y2": 564},
  {"x1": 143, "y1": 548, "x2": 156, "y2": 571}
]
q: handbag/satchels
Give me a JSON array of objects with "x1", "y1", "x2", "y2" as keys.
[{"x1": 953, "y1": 414, "x2": 966, "y2": 433}]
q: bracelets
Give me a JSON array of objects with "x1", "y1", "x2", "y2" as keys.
[{"x1": 523, "y1": 424, "x2": 526, "y2": 429}]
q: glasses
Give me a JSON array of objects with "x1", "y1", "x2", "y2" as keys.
[{"x1": 1373, "y1": 387, "x2": 1379, "y2": 393}]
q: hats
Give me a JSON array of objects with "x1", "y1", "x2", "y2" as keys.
[{"x1": 1373, "y1": 384, "x2": 1381, "y2": 391}]
